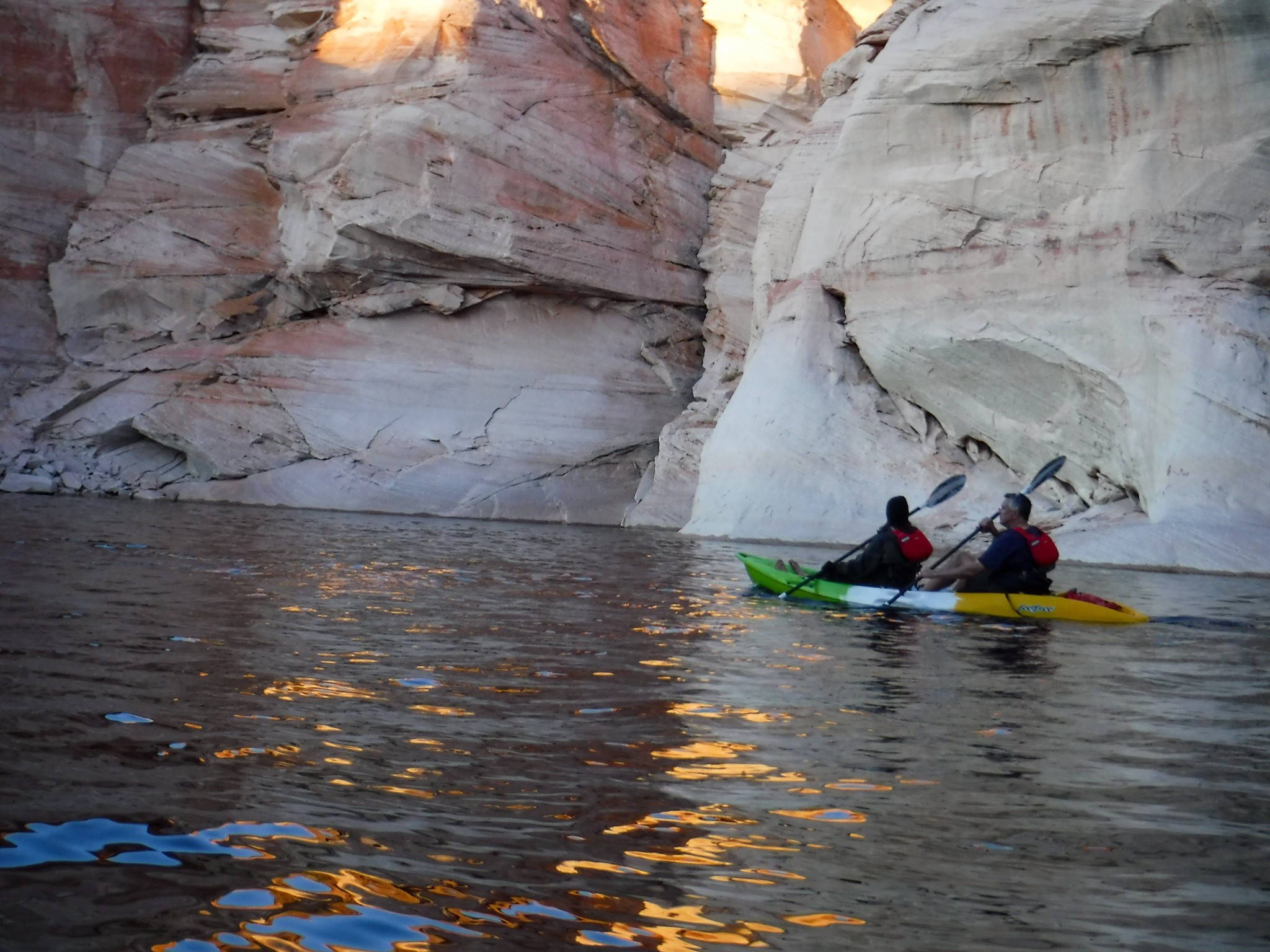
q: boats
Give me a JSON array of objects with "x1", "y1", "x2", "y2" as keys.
[{"x1": 736, "y1": 553, "x2": 1149, "y2": 623}]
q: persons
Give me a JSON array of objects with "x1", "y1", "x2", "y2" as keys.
[
  {"x1": 776, "y1": 496, "x2": 922, "y2": 589},
  {"x1": 920, "y1": 492, "x2": 1055, "y2": 595}
]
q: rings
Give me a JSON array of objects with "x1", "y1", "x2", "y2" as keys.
[{"x1": 918, "y1": 572, "x2": 921, "y2": 575}]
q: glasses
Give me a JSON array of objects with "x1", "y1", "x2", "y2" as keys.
[{"x1": 998, "y1": 508, "x2": 1008, "y2": 514}]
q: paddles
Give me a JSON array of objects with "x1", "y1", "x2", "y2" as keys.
[
  {"x1": 880, "y1": 455, "x2": 1066, "y2": 609},
  {"x1": 777, "y1": 474, "x2": 966, "y2": 600}
]
